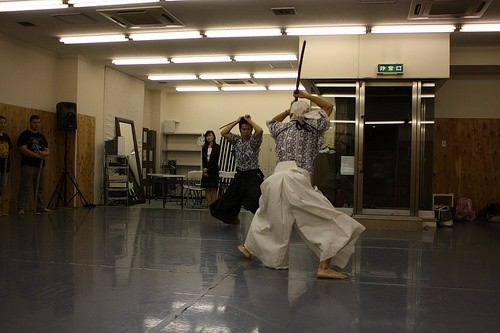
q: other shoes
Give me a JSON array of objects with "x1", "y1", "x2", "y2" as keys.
[
  {"x1": 17, "y1": 209, "x2": 24, "y2": 215},
  {"x1": 36, "y1": 208, "x2": 52, "y2": 212}
]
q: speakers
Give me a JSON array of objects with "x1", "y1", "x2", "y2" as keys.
[{"x1": 56, "y1": 101, "x2": 77, "y2": 131}]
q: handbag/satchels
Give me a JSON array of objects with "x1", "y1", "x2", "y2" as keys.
[{"x1": 434, "y1": 205, "x2": 453, "y2": 226}]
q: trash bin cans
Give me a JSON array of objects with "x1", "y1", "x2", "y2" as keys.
[{"x1": 154, "y1": 181, "x2": 164, "y2": 199}]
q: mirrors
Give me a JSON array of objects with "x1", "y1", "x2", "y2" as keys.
[{"x1": 115, "y1": 116, "x2": 145, "y2": 204}]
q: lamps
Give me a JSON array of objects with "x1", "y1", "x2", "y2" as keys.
[{"x1": 0, "y1": 0, "x2": 500, "y2": 93}]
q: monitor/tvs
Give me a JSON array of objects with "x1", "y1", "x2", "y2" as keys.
[{"x1": 107, "y1": 137, "x2": 125, "y2": 156}]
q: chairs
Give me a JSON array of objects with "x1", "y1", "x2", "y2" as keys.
[{"x1": 175, "y1": 171, "x2": 237, "y2": 209}]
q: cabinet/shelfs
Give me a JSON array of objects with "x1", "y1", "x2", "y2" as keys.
[
  {"x1": 104, "y1": 155, "x2": 130, "y2": 206},
  {"x1": 160, "y1": 130, "x2": 205, "y2": 198}
]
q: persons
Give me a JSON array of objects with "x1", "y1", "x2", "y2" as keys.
[
  {"x1": 209, "y1": 115, "x2": 264, "y2": 226},
  {"x1": 201, "y1": 130, "x2": 221, "y2": 205},
  {"x1": 17, "y1": 115, "x2": 52, "y2": 215},
  {"x1": 0, "y1": 115, "x2": 13, "y2": 217},
  {"x1": 239, "y1": 90, "x2": 366, "y2": 280}
]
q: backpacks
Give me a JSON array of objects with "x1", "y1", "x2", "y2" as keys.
[{"x1": 456, "y1": 198, "x2": 474, "y2": 221}]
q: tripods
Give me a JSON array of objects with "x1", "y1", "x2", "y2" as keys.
[{"x1": 46, "y1": 132, "x2": 90, "y2": 208}]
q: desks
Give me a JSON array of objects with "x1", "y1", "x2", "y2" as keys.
[{"x1": 147, "y1": 173, "x2": 185, "y2": 208}]
